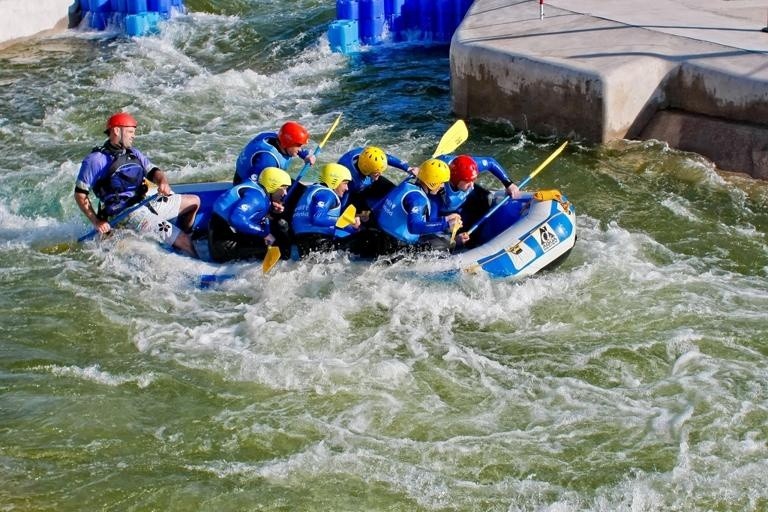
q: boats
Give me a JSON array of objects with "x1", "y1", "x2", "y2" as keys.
[{"x1": 102, "y1": 182, "x2": 577, "y2": 292}]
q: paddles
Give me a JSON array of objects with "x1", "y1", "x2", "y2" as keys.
[
  {"x1": 281, "y1": 112, "x2": 342, "y2": 206},
  {"x1": 337, "y1": 204, "x2": 360, "y2": 229},
  {"x1": 362, "y1": 119, "x2": 468, "y2": 218},
  {"x1": 450, "y1": 215, "x2": 461, "y2": 246},
  {"x1": 260, "y1": 218, "x2": 280, "y2": 276},
  {"x1": 467, "y1": 139, "x2": 569, "y2": 237}
]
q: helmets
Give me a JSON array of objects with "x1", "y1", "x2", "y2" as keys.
[
  {"x1": 279, "y1": 123, "x2": 307, "y2": 150},
  {"x1": 320, "y1": 164, "x2": 351, "y2": 192},
  {"x1": 106, "y1": 114, "x2": 136, "y2": 130},
  {"x1": 417, "y1": 159, "x2": 450, "y2": 191},
  {"x1": 259, "y1": 166, "x2": 291, "y2": 192},
  {"x1": 450, "y1": 155, "x2": 480, "y2": 184},
  {"x1": 359, "y1": 147, "x2": 387, "y2": 177}
]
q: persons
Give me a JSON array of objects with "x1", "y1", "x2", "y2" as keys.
[
  {"x1": 291, "y1": 162, "x2": 374, "y2": 261},
  {"x1": 74, "y1": 112, "x2": 201, "y2": 263},
  {"x1": 206, "y1": 166, "x2": 293, "y2": 264},
  {"x1": 335, "y1": 145, "x2": 421, "y2": 226},
  {"x1": 369, "y1": 159, "x2": 464, "y2": 263},
  {"x1": 232, "y1": 120, "x2": 318, "y2": 221},
  {"x1": 433, "y1": 153, "x2": 520, "y2": 253}
]
